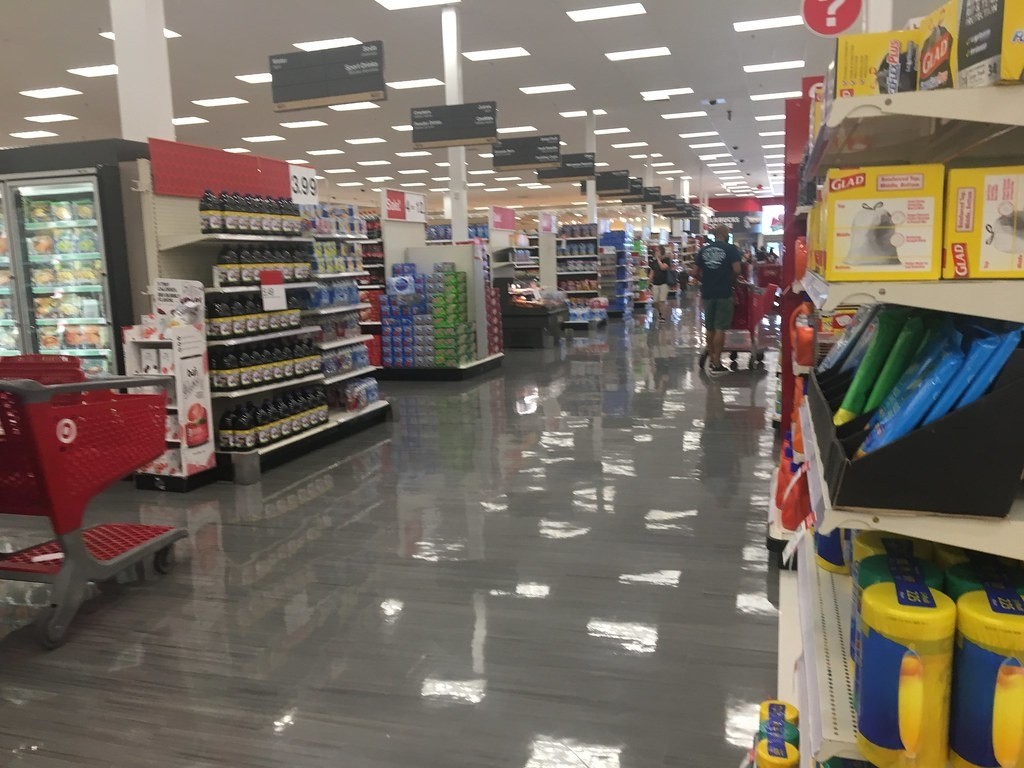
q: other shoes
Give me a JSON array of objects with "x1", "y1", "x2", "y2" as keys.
[
  {"x1": 708, "y1": 362, "x2": 731, "y2": 374},
  {"x1": 658, "y1": 317, "x2": 667, "y2": 323}
]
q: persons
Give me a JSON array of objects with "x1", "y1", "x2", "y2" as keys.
[
  {"x1": 647, "y1": 244, "x2": 671, "y2": 323},
  {"x1": 691, "y1": 223, "x2": 742, "y2": 374}
]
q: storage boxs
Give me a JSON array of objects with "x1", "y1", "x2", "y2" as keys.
[
  {"x1": 0, "y1": 353, "x2": 169, "y2": 519},
  {"x1": 730, "y1": 284, "x2": 765, "y2": 331}
]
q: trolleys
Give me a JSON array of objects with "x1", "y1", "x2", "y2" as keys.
[
  {"x1": 1, "y1": 353, "x2": 187, "y2": 648},
  {"x1": 698, "y1": 277, "x2": 783, "y2": 376}
]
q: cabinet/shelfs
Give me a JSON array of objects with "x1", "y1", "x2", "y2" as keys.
[
  {"x1": 359, "y1": 211, "x2": 505, "y2": 381},
  {"x1": 767, "y1": 0, "x2": 1024, "y2": 767},
  {"x1": 120, "y1": 138, "x2": 391, "y2": 495},
  {"x1": 425, "y1": 207, "x2": 705, "y2": 347}
]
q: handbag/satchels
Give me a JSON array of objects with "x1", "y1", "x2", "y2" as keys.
[{"x1": 665, "y1": 268, "x2": 680, "y2": 287}]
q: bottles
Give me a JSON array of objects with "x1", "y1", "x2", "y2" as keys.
[
  {"x1": 209, "y1": 333, "x2": 322, "y2": 389},
  {"x1": 361, "y1": 244, "x2": 383, "y2": 264},
  {"x1": 328, "y1": 377, "x2": 379, "y2": 413},
  {"x1": 323, "y1": 344, "x2": 369, "y2": 378},
  {"x1": 212, "y1": 242, "x2": 311, "y2": 287},
  {"x1": 218, "y1": 384, "x2": 331, "y2": 452},
  {"x1": 205, "y1": 290, "x2": 301, "y2": 338},
  {"x1": 200, "y1": 189, "x2": 301, "y2": 236},
  {"x1": 315, "y1": 310, "x2": 362, "y2": 342},
  {"x1": 358, "y1": 268, "x2": 384, "y2": 285},
  {"x1": 358, "y1": 210, "x2": 382, "y2": 239},
  {"x1": 311, "y1": 279, "x2": 358, "y2": 309}
]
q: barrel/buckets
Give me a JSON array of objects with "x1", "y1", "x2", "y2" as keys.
[
  {"x1": 788, "y1": 293, "x2": 816, "y2": 367},
  {"x1": 775, "y1": 374, "x2": 820, "y2": 531}
]
